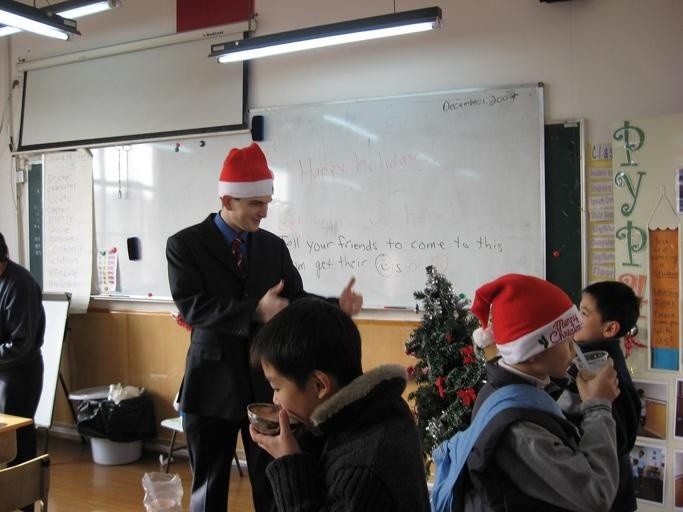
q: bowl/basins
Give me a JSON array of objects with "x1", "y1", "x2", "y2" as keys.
[{"x1": 245, "y1": 403, "x2": 300, "y2": 435}]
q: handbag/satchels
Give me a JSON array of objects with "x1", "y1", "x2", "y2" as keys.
[{"x1": 429, "y1": 430, "x2": 470, "y2": 512}]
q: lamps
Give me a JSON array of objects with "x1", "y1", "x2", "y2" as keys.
[
  {"x1": 0, "y1": 0, "x2": 123, "y2": 41},
  {"x1": 207, "y1": 0, "x2": 444, "y2": 63}
]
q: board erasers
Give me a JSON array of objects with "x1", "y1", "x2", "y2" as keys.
[{"x1": 128, "y1": 238, "x2": 138, "y2": 261}]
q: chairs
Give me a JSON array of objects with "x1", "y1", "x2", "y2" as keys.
[
  {"x1": 160, "y1": 391, "x2": 243, "y2": 480},
  {"x1": 0, "y1": 454, "x2": 50, "y2": 512}
]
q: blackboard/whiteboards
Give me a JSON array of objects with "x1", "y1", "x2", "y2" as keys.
[{"x1": 27, "y1": 81, "x2": 585, "y2": 315}]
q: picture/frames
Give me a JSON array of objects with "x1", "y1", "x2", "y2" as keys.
[{"x1": 628, "y1": 378, "x2": 683, "y2": 511}]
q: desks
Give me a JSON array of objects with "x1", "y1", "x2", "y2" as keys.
[{"x1": 0, "y1": 413, "x2": 33, "y2": 470}]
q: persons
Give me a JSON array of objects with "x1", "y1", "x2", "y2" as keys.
[
  {"x1": 249, "y1": 296, "x2": 433, "y2": 512},
  {"x1": 0, "y1": 232, "x2": 46, "y2": 511},
  {"x1": 551, "y1": 281, "x2": 641, "y2": 512},
  {"x1": 458, "y1": 273, "x2": 621, "y2": 512},
  {"x1": 166, "y1": 144, "x2": 363, "y2": 512}
]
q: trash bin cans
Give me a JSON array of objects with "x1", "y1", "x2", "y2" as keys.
[{"x1": 68, "y1": 385, "x2": 148, "y2": 466}]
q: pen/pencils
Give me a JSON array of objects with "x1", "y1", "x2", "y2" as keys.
[
  {"x1": 107, "y1": 294, "x2": 130, "y2": 297},
  {"x1": 383, "y1": 306, "x2": 409, "y2": 311}
]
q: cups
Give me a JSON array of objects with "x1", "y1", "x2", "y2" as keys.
[
  {"x1": 146, "y1": 499, "x2": 179, "y2": 512},
  {"x1": 571, "y1": 354, "x2": 608, "y2": 382}
]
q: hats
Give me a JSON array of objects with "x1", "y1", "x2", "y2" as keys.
[
  {"x1": 471, "y1": 274, "x2": 584, "y2": 365},
  {"x1": 218, "y1": 143, "x2": 273, "y2": 199}
]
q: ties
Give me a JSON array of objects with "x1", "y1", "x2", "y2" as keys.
[{"x1": 230, "y1": 237, "x2": 246, "y2": 278}]
